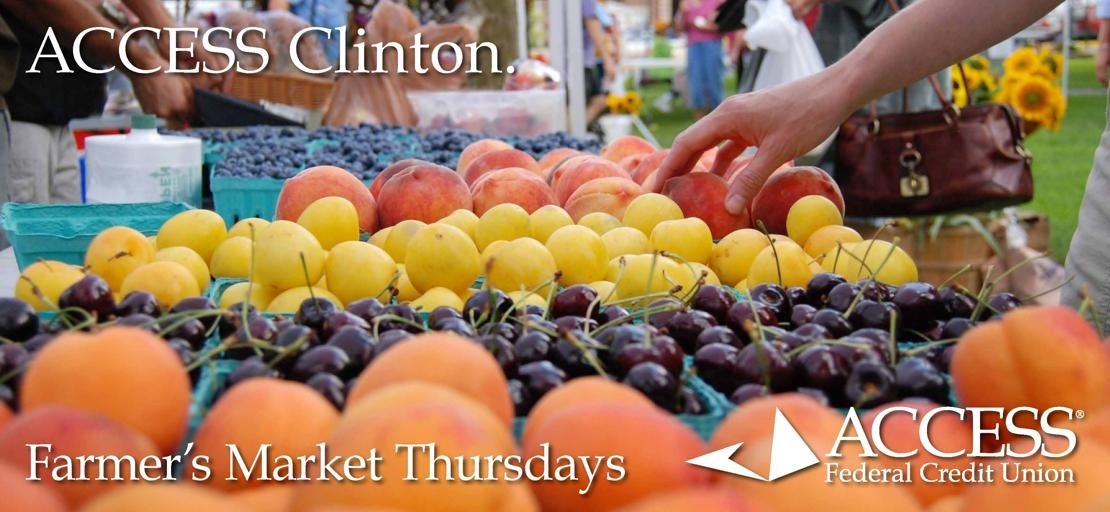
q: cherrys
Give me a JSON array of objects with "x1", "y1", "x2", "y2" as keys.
[{"x1": 1, "y1": 270, "x2": 1035, "y2": 417}]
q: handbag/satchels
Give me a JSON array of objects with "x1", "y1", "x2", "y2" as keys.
[{"x1": 835, "y1": 104, "x2": 1033, "y2": 217}]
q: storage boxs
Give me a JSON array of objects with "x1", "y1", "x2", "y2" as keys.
[{"x1": 403, "y1": 90, "x2": 568, "y2": 140}]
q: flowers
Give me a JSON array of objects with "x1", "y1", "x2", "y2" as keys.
[
  {"x1": 606, "y1": 91, "x2": 643, "y2": 116},
  {"x1": 912, "y1": 44, "x2": 1070, "y2": 256}
]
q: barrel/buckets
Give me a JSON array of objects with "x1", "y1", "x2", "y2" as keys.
[{"x1": 85, "y1": 114, "x2": 203, "y2": 208}]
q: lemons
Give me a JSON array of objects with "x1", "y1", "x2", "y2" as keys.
[{"x1": 14, "y1": 194, "x2": 921, "y2": 321}]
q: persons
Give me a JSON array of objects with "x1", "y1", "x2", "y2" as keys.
[
  {"x1": 106, "y1": 0, "x2": 968, "y2": 167},
  {"x1": 1, "y1": 0, "x2": 235, "y2": 246},
  {"x1": 1092, "y1": 1, "x2": 1110, "y2": 84},
  {"x1": 648, "y1": 1, "x2": 1110, "y2": 347}
]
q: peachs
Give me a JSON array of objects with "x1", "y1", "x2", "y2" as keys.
[
  {"x1": 1, "y1": 284, "x2": 1109, "y2": 511},
  {"x1": 275, "y1": 136, "x2": 849, "y2": 241}
]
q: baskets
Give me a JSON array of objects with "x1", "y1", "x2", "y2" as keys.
[{"x1": 201, "y1": 65, "x2": 336, "y2": 115}]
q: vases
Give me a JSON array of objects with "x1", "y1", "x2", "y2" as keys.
[
  {"x1": 867, "y1": 215, "x2": 1053, "y2": 305},
  {"x1": 597, "y1": 116, "x2": 633, "y2": 146}
]
